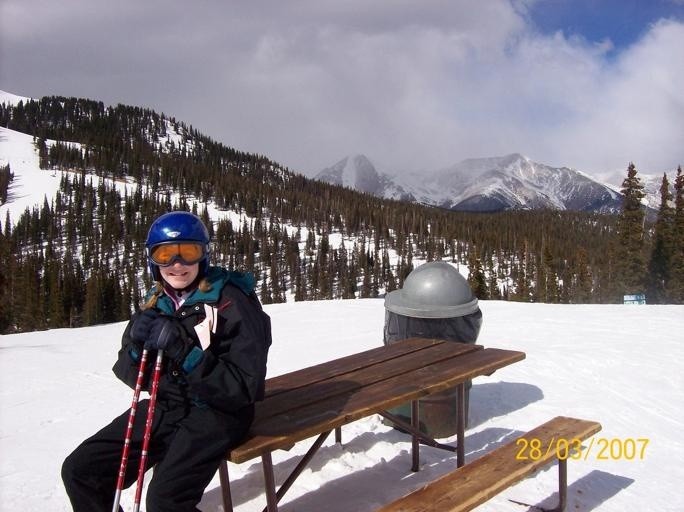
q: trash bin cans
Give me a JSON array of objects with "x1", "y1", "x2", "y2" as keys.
[{"x1": 382, "y1": 261, "x2": 484, "y2": 438}]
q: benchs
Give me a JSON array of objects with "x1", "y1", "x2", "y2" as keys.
[{"x1": 377, "y1": 416, "x2": 606, "y2": 512}]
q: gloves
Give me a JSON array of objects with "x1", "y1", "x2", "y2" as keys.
[{"x1": 130, "y1": 310, "x2": 197, "y2": 368}]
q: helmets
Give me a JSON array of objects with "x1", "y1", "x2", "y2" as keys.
[{"x1": 145, "y1": 211, "x2": 209, "y2": 248}]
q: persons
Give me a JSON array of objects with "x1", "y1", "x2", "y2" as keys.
[{"x1": 62, "y1": 211, "x2": 272, "y2": 512}]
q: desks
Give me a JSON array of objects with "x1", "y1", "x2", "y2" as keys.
[{"x1": 219, "y1": 334, "x2": 527, "y2": 511}]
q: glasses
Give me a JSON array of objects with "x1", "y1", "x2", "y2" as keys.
[{"x1": 148, "y1": 242, "x2": 208, "y2": 266}]
